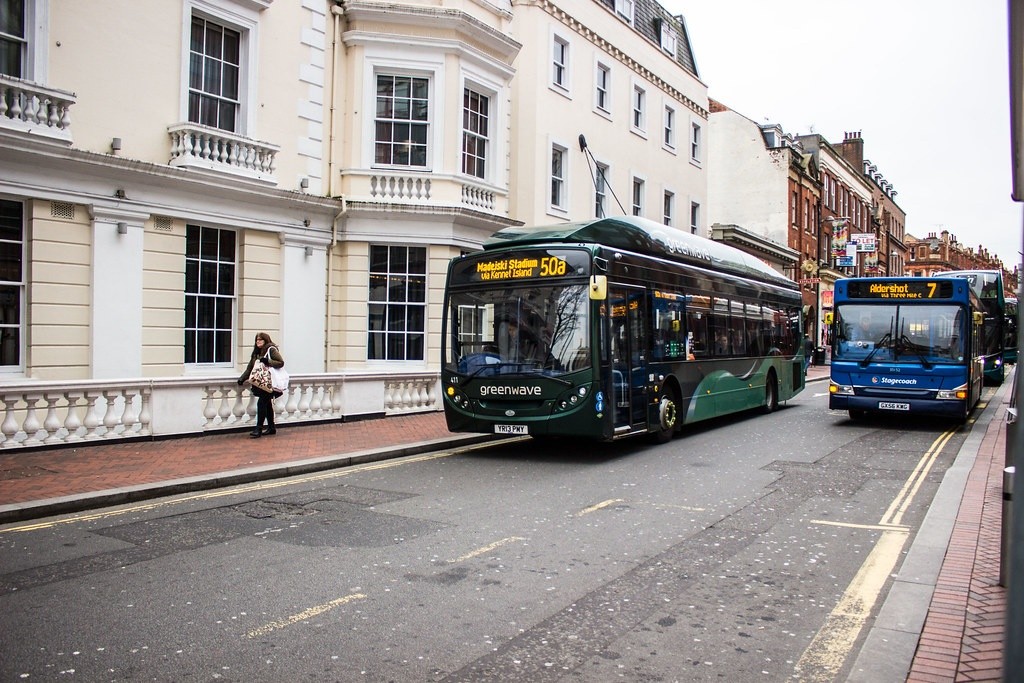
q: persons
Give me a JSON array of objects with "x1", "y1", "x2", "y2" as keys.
[
  {"x1": 507, "y1": 324, "x2": 524, "y2": 351},
  {"x1": 851, "y1": 317, "x2": 880, "y2": 342},
  {"x1": 237, "y1": 333, "x2": 285, "y2": 437},
  {"x1": 804, "y1": 334, "x2": 814, "y2": 375},
  {"x1": 699, "y1": 331, "x2": 736, "y2": 356}
]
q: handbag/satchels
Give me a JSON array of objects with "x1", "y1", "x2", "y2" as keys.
[
  {"x1": 267, "y1": 347, "x2": 289, "y2": 389},
  {"x1": 248, "y1": 346, "x2": 278, "y2": 393}
]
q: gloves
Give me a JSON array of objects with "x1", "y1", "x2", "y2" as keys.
[
  {"x1": 260, "y1": 358, "x2": 267, "y2": 366},
  {"x1": 238, "y1": 380, "x2": 243, "y2": 385}
]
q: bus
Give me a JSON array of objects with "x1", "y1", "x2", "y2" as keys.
[
  {"x1": 829, "y1": 275, "x2": 985, "y2": 421},
  {"x1": 441, "y1": 215, "x2": 806, "y2": 444},
  {"x1": 931, "y1": 268, "x2": 1006, "y2": 385},
  {"x1": 1004, "y1": 298, "x2": 1017, "y2": 364}
]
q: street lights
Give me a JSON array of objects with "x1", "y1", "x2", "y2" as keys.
[
  {"x1": 817, "y1": 216, "x2": 834, "y2": 348},
  {"x1": 886, "y1": 252, "x2": 898, "y2": 276}
]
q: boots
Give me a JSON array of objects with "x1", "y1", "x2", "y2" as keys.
[
  {"x1": 262, "y1": 422, "x2": 276, "y2": 435},
  {"x1": 250, "y1": 424, "x2": 262, "y2": 436}
]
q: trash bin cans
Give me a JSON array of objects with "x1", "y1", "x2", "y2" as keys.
[{"x1": 813, "y1": 346, "x2": 826, "y2": 365}]
